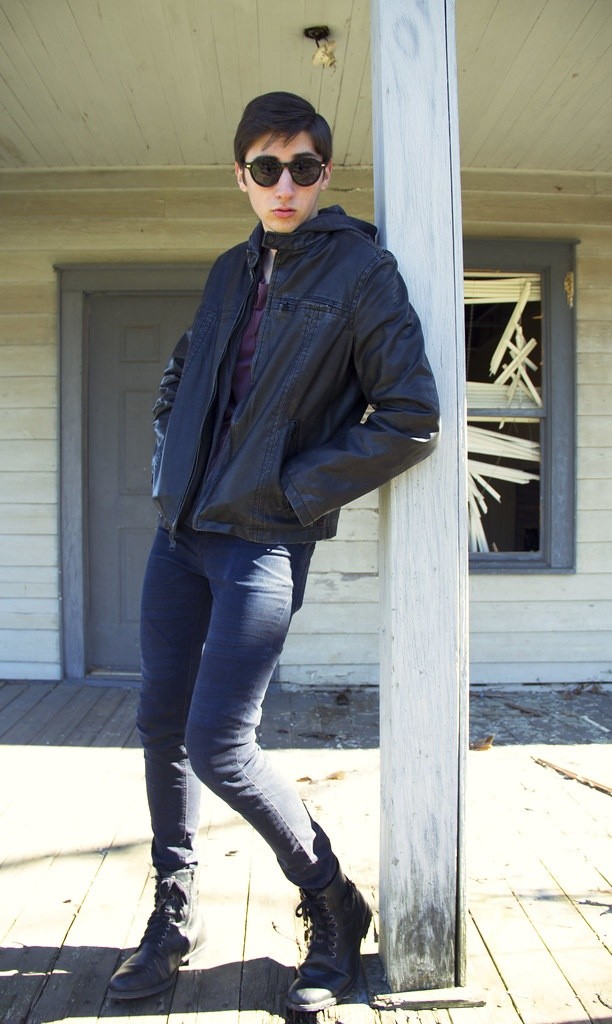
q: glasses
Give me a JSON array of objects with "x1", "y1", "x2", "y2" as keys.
[{"x1": 243, "y1": 155, "x2": 327, "y2": 188}]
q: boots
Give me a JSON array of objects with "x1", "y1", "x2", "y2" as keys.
[
  {"x1": 106, "y1": 866, "x2": 208, "y2": 999},
  {"x1": 284, "y1": 853, "x2": 372, "y2": 1012}
]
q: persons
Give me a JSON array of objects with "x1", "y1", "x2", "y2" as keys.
[{"x1": 110, "y1": 91, "x2": 440, "y2": 1011}]
